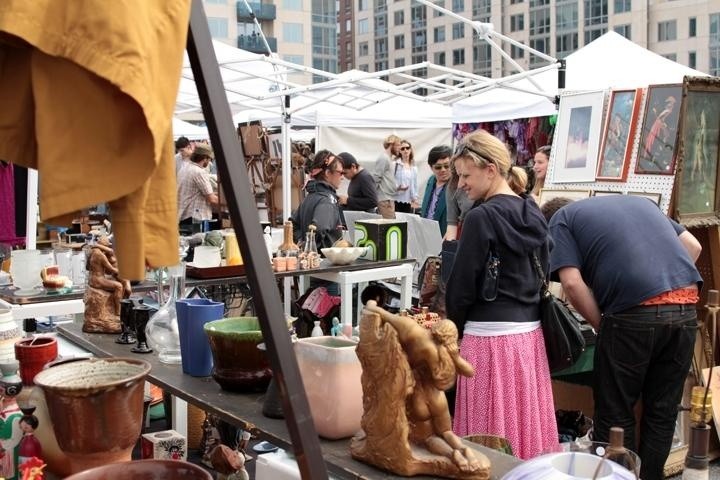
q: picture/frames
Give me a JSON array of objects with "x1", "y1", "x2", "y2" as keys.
[
  {"x1": 238, "y1": 120, "x2": 268, "y2": 161},
  {"x1": 551, "y1": 90, "x2": 606, "y2": 184},
  {"x1": 634, "y1": 83, "x2": 685, "y2": 175},
  {"x1": 662, "y1": 321, "x2": 720, "y2": 477},
  {"x1": 668, "y1": 75, "x2": 720, "y2": 230},
  {"x1": 592, "y1": 190, "x2": 622, "y2": 197},
  {"x1": 537, "y1": 188, "x2": 591, "y2": 210},
  {"x1": 626, "y1": 192, "x2": 662, "y2": 207},
  {"x1": 595, "y1": 87, "x2": 642, "y2": 182}
]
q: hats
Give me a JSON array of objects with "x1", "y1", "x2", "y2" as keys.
[{"x1": 193, "y1": 142, "x2": 216, "y2": 160}]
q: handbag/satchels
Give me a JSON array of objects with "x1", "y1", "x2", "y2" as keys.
[
  {"x1": 529, "y1": 246, "x2": 586, "y2": 373},
  {"x1": 439, "y1": 239, "x2": 500, "y2": 302}
]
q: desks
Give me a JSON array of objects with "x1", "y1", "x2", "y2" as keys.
[{"x1": 0, "y1": 258, "x2": 527, "y2": 480}]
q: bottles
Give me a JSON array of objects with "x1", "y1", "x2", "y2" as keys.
[
  {"x1": 301, "y1": 231, "x2": 319, "y2": 269},
  {"x1": 146, "y1": 261, "x2": 186, "y2": 366}
]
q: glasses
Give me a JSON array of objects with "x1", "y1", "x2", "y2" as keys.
[
  {"x1": 542, "y1": 145, "x2": 551, "y2": 160},
  {"x1": 400, "y1": 147, "x2": 410, "y2": 150},
  {"x1": 454, "y1": 143, "x2": 495, "y2": 164},
  {"x1": 432, "y1": 164, "x2": 450, "y2": 170},
  {"x1": 330, "y1": 169, "x2": 346, "y2": 175}
]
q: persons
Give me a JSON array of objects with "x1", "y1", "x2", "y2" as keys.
[
  {"x1": 288, "y1": 148, "x2": 348, "y2": 258},
  {"x1": 445, "y1": 128, "x2": 563, "y2": 462},
  {"x1": 439, "y1": 167, "x2": 477, "y2": 242},
  {"x1": 174, "y1": 135, "x2": 193, "y2": 175},
  {"x1": 420, "y1": 145, "x2": 453, "y2": 238},
  {"x1": 529, "y1": 144, "x2": 552, "y2": 195},
  {"x1": 176, "y1": 143, "x2": 219, "y2": 226},
  {"x1": 336, "y1": 151, "x2": 378, "y2": 214},
  {"x1": 88, "y1": 235, "x2": 132, "y2": 316},
  {"x1": 540, "y1": 193, "x2": 704, "y2": 479},
  {"x1": 371, "y1": 133, "x2": 402, "y2": 219},
  {"x1": 365, "y1": 300, "x2": 481, "y2": 475},
  {"x1": 391, "y1": 139, "x2": 420, "y2": 213}
]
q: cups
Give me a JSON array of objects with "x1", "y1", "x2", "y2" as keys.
[
  {"x1": 273, "y1": 256, "x2": 286, "y2": 273},
  {"x1": 192, "y1": 245, "x2": 220, "y2": 267},
  {"x1": 285, "y1": 256, "x2": 298, "y2": 271},
  {"x1": 222, "y1": 227, "x2": 243, "y2": 266},
  {"x1": 176, "y1": 298, "x2": 225, "y2": 377},
  {"x1": 40, "y1": 246, "x2": 86, "y2": 291}
]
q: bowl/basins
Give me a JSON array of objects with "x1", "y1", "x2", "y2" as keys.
[{"x1": 319, "y1": 246, "x2": 368, "y2": 265}]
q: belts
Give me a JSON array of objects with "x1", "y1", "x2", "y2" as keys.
[{"x1": 618, "y1": 304, "x2": 696, "y2": 315}]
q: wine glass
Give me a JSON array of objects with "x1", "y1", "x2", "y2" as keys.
[{"x1": 113, "y1": 297, "x2": 151, "y2": 354}]
